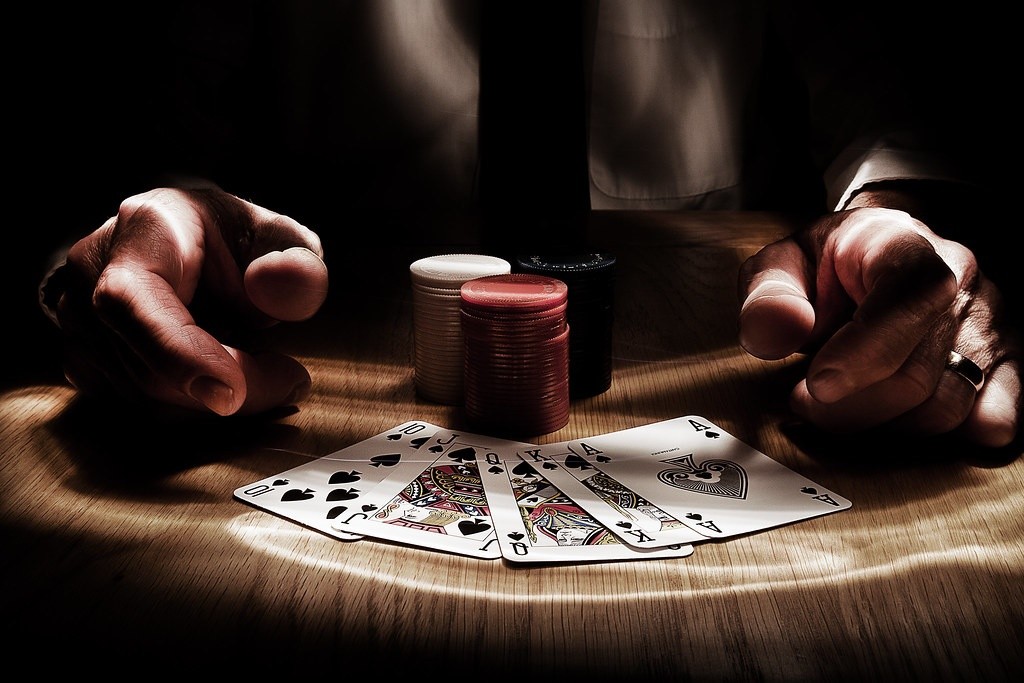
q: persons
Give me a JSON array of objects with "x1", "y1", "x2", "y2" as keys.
[{"x1": 1, "y1": 0, "x2": 1024, "y2": 447}]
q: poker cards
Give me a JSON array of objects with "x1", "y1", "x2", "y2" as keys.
[{"x1": 230, "y1": 414, "x2": 853, "y2": 566}]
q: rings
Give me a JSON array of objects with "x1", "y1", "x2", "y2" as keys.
[{"x1": 946, "y1": 348, "x2": 985, "y2": 395}]
